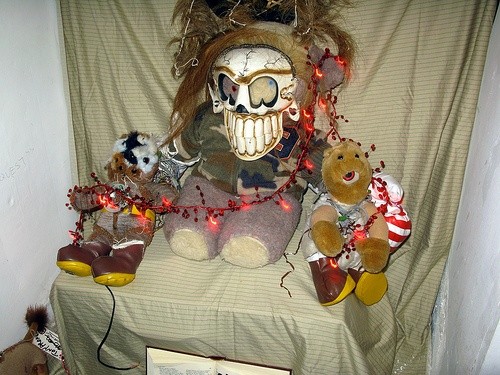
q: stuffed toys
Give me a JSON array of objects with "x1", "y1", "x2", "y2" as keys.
[
  {"x1": 158, "y1": 0, "x2": 350, "y2": 271},
  {"x1": 1, "y1": 306, "x2": 50, "y2": 375},
  {"x1": 301, "y1": 136, "x2": 391, "y2": 308},
  {"x1": 56, "y1": 131, "x2": 180, "y2": 287}
]
